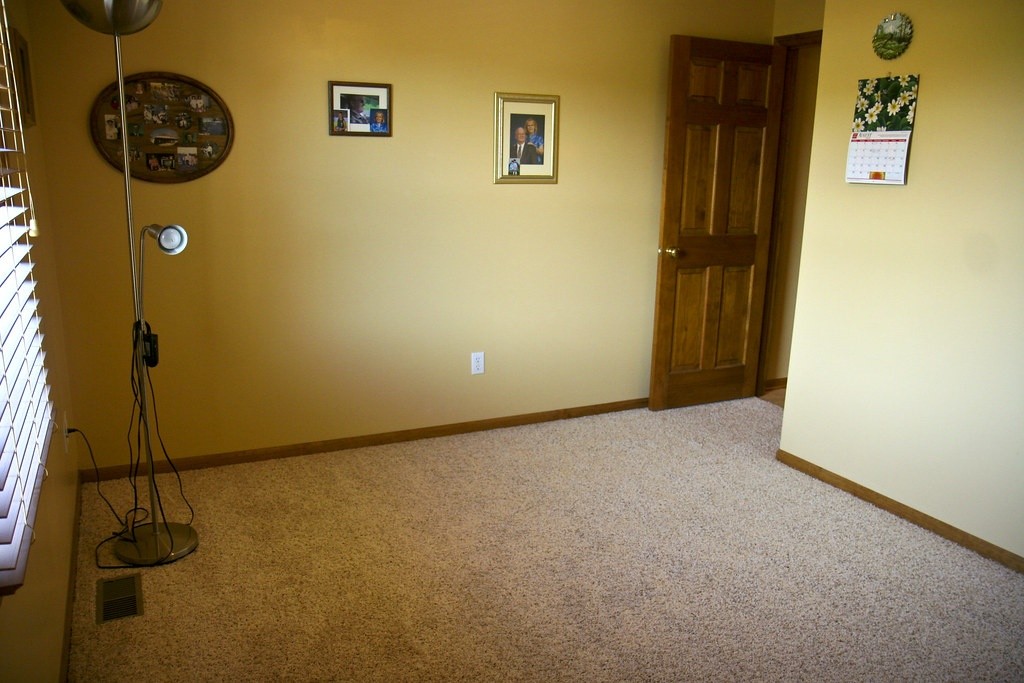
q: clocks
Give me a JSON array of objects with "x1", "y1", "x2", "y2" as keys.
[{"x1": 871, "y1": 13, "x2": 913, "y2": 59}]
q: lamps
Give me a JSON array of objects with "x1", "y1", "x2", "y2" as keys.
[{"x1": 60, "y1": 0, "x2": 199, "y2": 566}]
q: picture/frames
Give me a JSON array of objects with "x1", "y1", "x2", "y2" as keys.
[
  {"x1": 494, "y1": 92, "x2": 560, "y2": 184},
  {"x1": 328, "y1": 81, "x2": 392, "y2": 137},
  {"x1": 90, "y1": 72, "x2": 235, "y2": 184},
  {"x1": 7, "y1": 25, "x2": 37, "y2": 127}
]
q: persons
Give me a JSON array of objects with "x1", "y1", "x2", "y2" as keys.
[
  {"x1": 332, "y1": 93, "x2": 389, "y2": 134},
  {"x1": 512, "y1": 118, "x2": 543, "y2": 163}
]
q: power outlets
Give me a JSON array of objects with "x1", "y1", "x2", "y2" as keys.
[{"x1": 471, "y1": 352, "x2": 484, "y2": 375}]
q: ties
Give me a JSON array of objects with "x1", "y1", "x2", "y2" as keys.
[{"x1": 517, "y1": 145, "x2": 522, "y2": 158}]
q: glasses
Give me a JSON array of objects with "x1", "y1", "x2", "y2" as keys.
[{"x1": 354, "y1": 99, "x2": 364, "y2": 104}]
